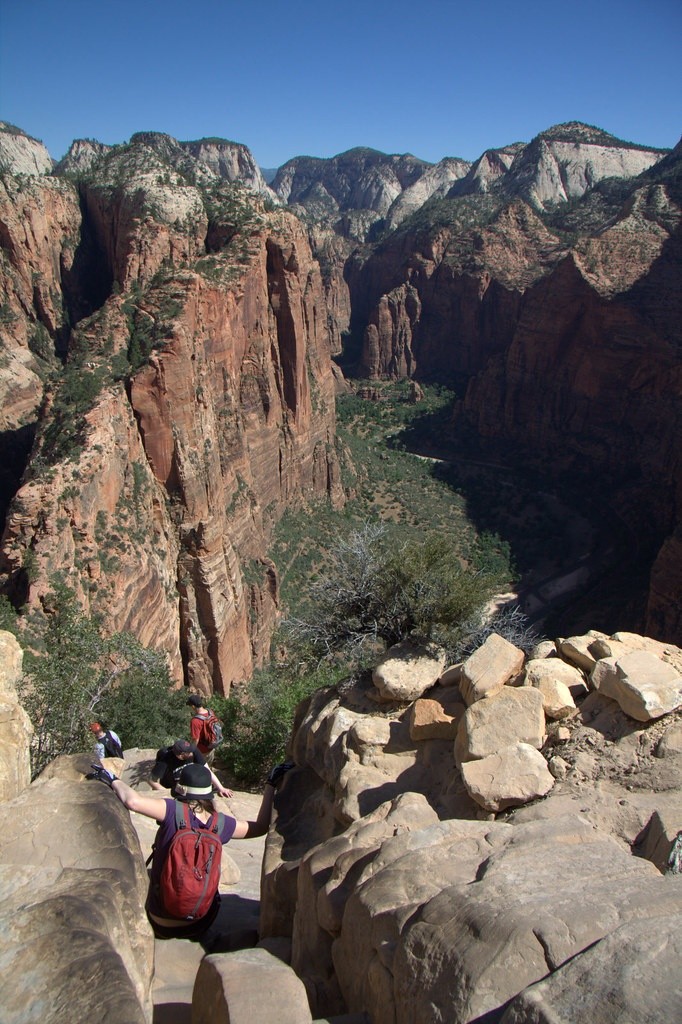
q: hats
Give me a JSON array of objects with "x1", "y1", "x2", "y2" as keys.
[
  {"x1": 171, "y1": 764, "x2": 214, "y2": 800},
  {"x1": 186, "y1": 695, "x2": 203, "y2": 706},
  {"x1": 91, "y1": 723, "x2": 102, "y2": 733},
  {"x1": 174, "y1": 739, "x2": 192, "y2": 759}
]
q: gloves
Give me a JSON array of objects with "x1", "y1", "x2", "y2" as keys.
[
  {"x1": 265, "y1": 761, "x2": 296, "y2": 788},
  {"x1": 85, "y1": 765, "x2": 119, "y2": 786}
]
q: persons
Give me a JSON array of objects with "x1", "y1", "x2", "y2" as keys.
[
  {"x1": 90, "y1": 723, "x2": 123, "y2": 759},
  {"x1": 148, "y1": 740, "x2": 234, "y2": 798},
  {"x1": 186, "y1": 694, "x2": 218, "y2": 768},
  {"x1": 85, "y1": 761, "x2": 295, "y2": 943}
]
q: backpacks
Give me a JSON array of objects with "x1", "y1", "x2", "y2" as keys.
[
  {"x1": 155, "y1": 746, "x2": 172, "y2": 761},
  {"x1": 98, "y1": 731, "x2": 124, "y2": 759},
  {"x1": 161, "y1": 801, "x2": 225, "y2": 921},
  {"x1": 194, "y1": 707, "x2": 224, "y2": 750}
]
